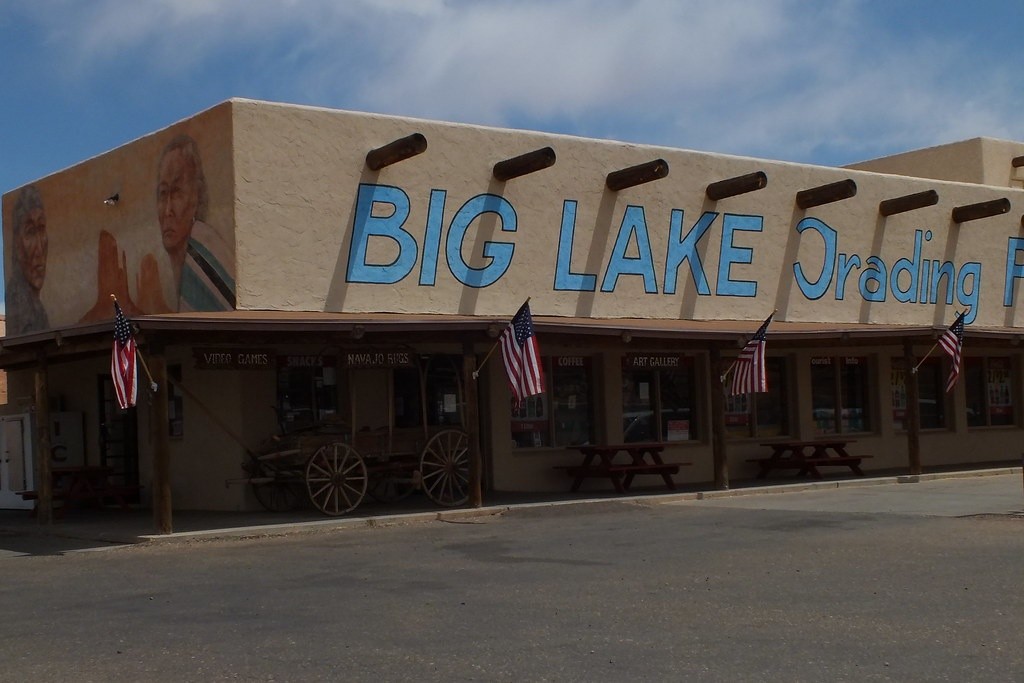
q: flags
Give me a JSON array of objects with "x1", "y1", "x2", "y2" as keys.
[
  {"x1": 111, "y1": 300, "x2": 141, "y2": 408},
  {"x1": 728, "y1": 313, "x2": 775, "y2": 397},
  {"x1": 499, "y1": 300, "x2": 545, "y2": 401},
  {"x1": 938, "y1": 313, "x2": 965, "y2": 392}
]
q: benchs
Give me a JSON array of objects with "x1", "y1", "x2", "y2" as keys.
[
  {"x1": 14, "y1": 483, "x2": 130, "y2": 502},
  {"x1": 746, "y1": 456, "x2": 862, "y2": 470},
  {"x1": 570, "y1": 464, "x2": 679, "y2": 478}
]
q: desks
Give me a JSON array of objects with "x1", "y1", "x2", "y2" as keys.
[
  {"x1": 743, "y1": 439, "x2": 865, "y2": 488},
  {"x1": 47, "y1": 465, "x2": 130, "y2": 517},
  {"x1": 566, "y1": 443, "x2": 678, "y2": 497}
]
